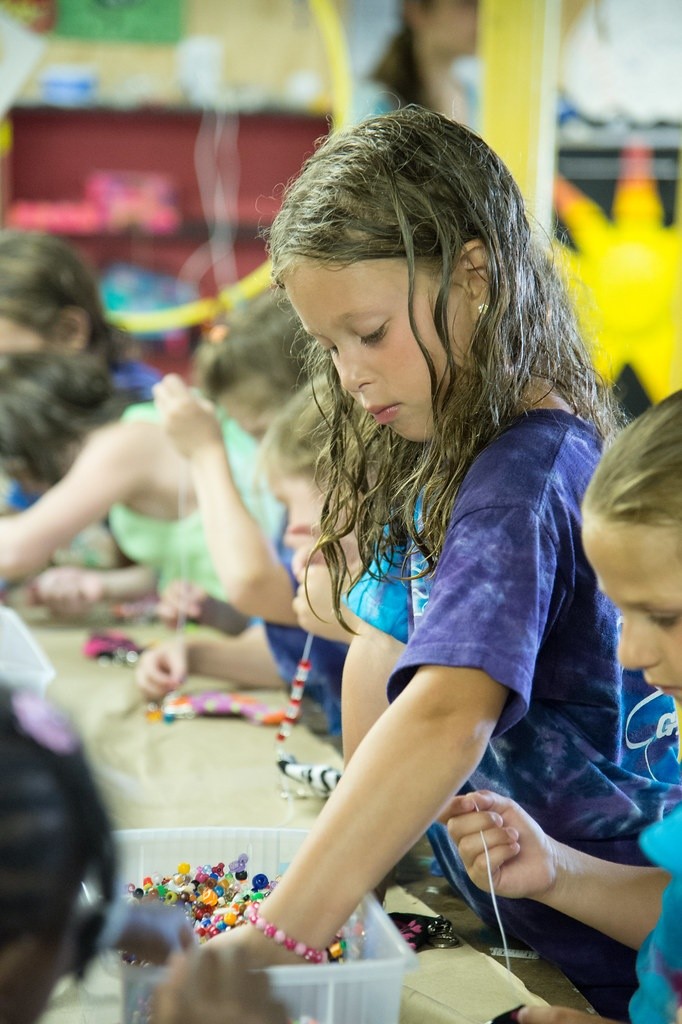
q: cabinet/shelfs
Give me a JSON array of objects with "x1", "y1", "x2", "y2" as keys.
[{"x1": 1, "y1": 103, "x2": 341, "y2": 378}]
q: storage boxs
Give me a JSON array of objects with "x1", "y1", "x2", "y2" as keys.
[{"x1": 78, "y1": 823, "x2": 419, "y2": 1024}]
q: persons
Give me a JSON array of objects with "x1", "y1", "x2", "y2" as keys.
[
  {"x1": 347, "y1": 0, "x2": 477, "y2": 131},
  {"x1": 201, "y1": 103, "x2": 682, "y2": 1023},
  {"x1": 438, "y1": 386, "x2": 681, "y2": 1023},
  {"x1": 0, "y1": 604, "x2": 294, "y2": 1024},
  {"x1": 0, "y1": 225, "x2": 373, "y2": 755}
]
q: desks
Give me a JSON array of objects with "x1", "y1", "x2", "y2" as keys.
[{"x1": 0, "y1": 585, "x2": 602, "y2": 1023}]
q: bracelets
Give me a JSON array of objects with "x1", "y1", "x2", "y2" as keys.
[{"x1": 244, "y1": 902, "x2": 328, "y2": 963}]
